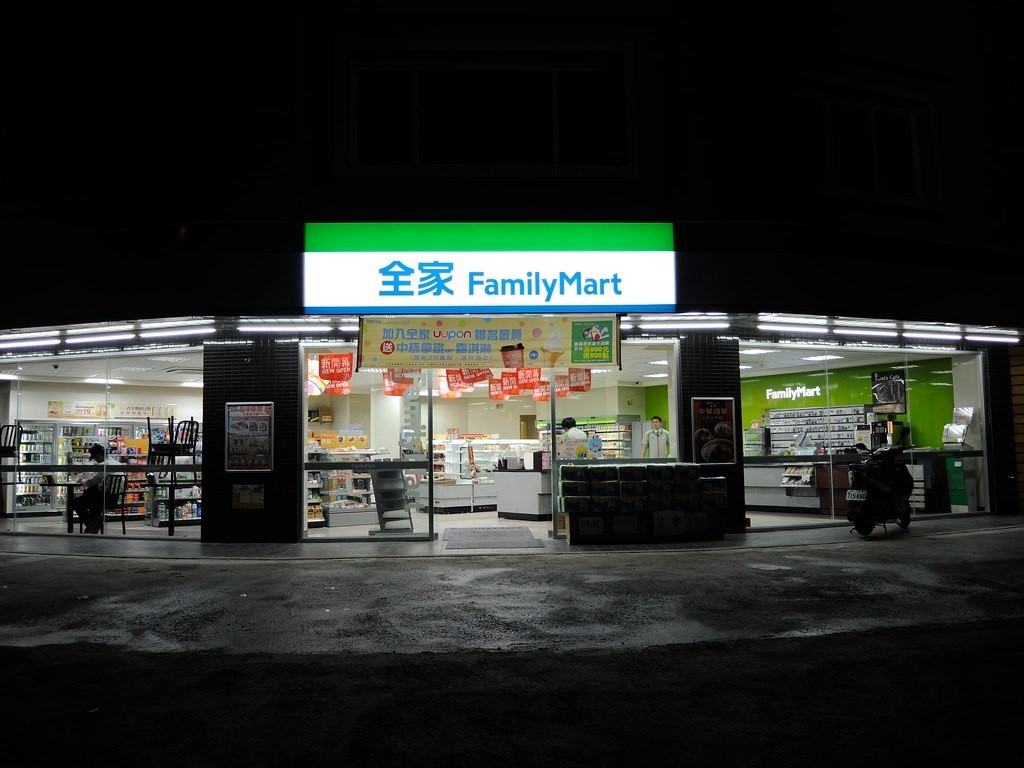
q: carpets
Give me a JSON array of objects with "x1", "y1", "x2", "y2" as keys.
[{"x1": 442, "y1": 527, "x2": 546, "y2": 550}]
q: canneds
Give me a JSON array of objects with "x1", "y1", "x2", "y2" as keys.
[
  {"x1": 16, "y1": 433, "x2": 44, "y2": 493},
  {"x1": 98, "y1": 428, "x2": 121, "y2": 435},
  {"x1": 57, "y1": 427, "x2": 72, "y2": 506}
]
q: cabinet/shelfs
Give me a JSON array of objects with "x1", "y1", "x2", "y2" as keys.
[
  {"x1": 145, "y1": 429, "x2": 446, "y2": 529},
  {"x1": 769, "y1": 404, "x2": 876, "y2": 455}
]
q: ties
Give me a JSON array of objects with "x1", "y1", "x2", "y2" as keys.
[{"x1": 657, "y1": 431, "x2": 659, "y2": 437}]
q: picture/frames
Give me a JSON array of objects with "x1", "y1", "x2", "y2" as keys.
[{"x1": 225, "y1": 401, "x2": 274, "y2": 473}]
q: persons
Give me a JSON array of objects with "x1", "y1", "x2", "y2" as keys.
[
  {"x1": 560, "y1": 418, "x2": 588, "y2": 441},
  {"x1": 72, "y1": 443, "x2": 124, "y2": 533},
  {"x1": 641, "y1": 416, "x2": 673, "y2": 458}
]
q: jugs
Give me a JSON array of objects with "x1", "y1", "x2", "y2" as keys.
[{"x1": 192, "y1": 486, "x2": 201, "y2": 498}]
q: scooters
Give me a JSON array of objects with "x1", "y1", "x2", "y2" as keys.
[{"x1": 843, "y1": 427, "x2": 916, "y2": 535}]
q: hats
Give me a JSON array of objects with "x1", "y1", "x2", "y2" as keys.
[{"x1": 88, "y1": 443, "x2": 104, "y2": 461}]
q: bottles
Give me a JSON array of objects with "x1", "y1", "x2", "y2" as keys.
[
  {"x1": 97, "y1": 426, "x2": 122, "y2": 436},
  {"x1": 158, "y1": 501, "x2": 201, "y2": 520},
  {"x1": 121, "y1": 445, "x2": 127, "y2": 453},
  {"x1": 156, "y1": 487, "x2": 166, "y2": 498}
]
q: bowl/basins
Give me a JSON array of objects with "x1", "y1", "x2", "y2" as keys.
[
  {"x1": 694, "y1": 425, "x2": 715, "y2": 442},
  {"x1": 714, "y1": 421, "x2": 733, "y2": 436},
  {"x1": 701, "y1": 438, "x2": 734, "y2": 463}
]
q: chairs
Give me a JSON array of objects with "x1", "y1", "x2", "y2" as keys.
[
  {"x1": 150, "y1": 420, "x2": 200, "y2": 481},
  {"x1": 79, "y1": 475, "x2": 128, "y2": 535},
  {"x1": 0, "y1": 425, "x2": 23, "y2": 482}
]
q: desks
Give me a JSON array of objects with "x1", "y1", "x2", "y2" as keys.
[
  {"x1": 139, "y1": 482, "x2": 201, "y2": 536},
  {"x1": 0, "y1": 482, "x2": 25, "y2": 531},
  {"x1": 39, "y1": 482, "x2": 88, "y2": 533}
]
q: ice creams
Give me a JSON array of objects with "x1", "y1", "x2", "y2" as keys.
[{"x1": 540, "y1": 327, "x2": 565, "y2": 367}]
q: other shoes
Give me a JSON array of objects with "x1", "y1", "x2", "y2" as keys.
[{"x1": 85, "y1": 516, "x2": 103, "y2": 534}]
